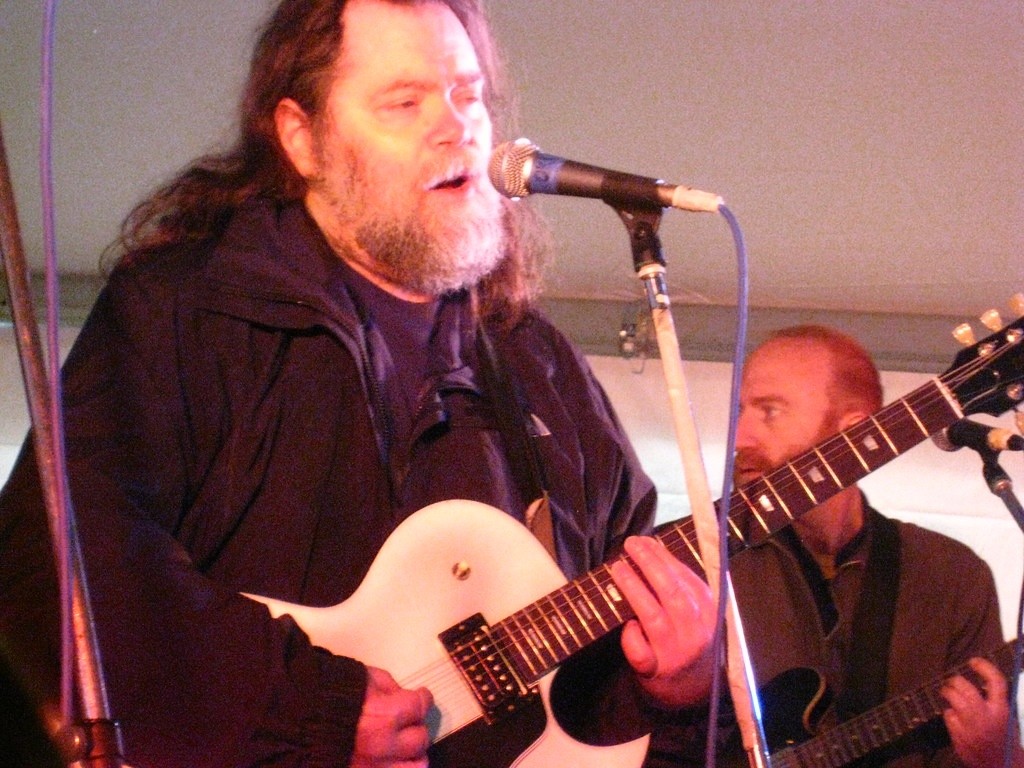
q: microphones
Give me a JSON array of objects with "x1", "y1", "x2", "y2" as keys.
[
  {"x1": 932, "y1": 418, "x2": 1024, "y2": 451},
  {"x1": 489, "y1": 138, "x2": 723, "y2": 210}
]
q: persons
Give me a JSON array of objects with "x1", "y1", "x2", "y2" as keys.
[
  {"x1": 641, "y1": 325, "x2": 1024, "y2": 768},
  {"x1": 0, "y1": 0, "x2": 722, "y2": 768}
]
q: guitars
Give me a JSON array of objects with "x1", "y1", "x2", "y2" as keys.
[
  {"x1": 646, "y1": 635, "x2": 1022, "y2": 767},
  {"x1": 226, "y1": 294, "x2": 1023, "y2": 767}
]
